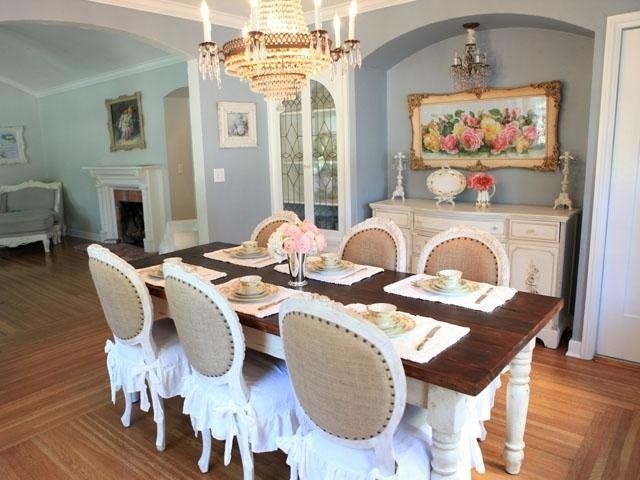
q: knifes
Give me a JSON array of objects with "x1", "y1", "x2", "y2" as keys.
[
  {"x1": 338, "y1": 267, "x2": 368, "y2": 280},
  {"x1": 255, "y1": 257, "x2": 273, "y2": 264},
  {"x1": 473, "y1": 286, "x2": 494, "y2": 304},
  {"x1": 416, "y1": 325, "x2": 440, "y2": 352},
  {"x1": 256, "y1": 298, "x2": 288, "y2": 311}
]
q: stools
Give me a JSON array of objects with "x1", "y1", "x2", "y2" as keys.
[{"x1": 0, "y1": 208, "x2": 63, "y2": 256}]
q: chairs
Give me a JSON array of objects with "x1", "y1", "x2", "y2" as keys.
[
  {"x1": 0, "y1": 178, "x2": 69, "y2": 244},
  {"x1": 84, "y1": 239, "x2": 189, "y2": 455},
  {"x1": 247, "y1": 207, "x2": 306, "y2": 254},
  {"x1": 275, "y1": 295, "x2": 486, "y2": 480},
  {"x1": 403, "y1": 224, "x2": 512, "y2": 423},
  {"x1": 159, "y1": 257, "x2": 301, "y2": 480},
  {"x1": 337, "y1": 216, "x2": 409, "y2": 275}
]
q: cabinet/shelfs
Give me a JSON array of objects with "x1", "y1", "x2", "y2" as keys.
[
  {"x1": 265, "y1": 75, "x2": 389, "y2": 257},
  {"x1": 365, "y1": 192, "x2": 589, "y2": 352}
]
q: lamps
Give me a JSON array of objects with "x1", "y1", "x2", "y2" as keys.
[
  {"x1": 195, "y1": 0, "x2": 361, "y2": 106},
  {"x1": 447, "y1": 19, "x2": 497, "y2": 99}
]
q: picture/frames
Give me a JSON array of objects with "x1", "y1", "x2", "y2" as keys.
[
  {"x1": 0, "y1": 125, "x2": 29, "y2": 166},
  {"x1": 215, "y1": 100, "x2": 260, "y2": 151},
  {"x1": 408, "y1": 78, "x2": 565, "y2": 171},
  {"x1": 104, "y1": 91, "x2": 146, "y2": 152}
]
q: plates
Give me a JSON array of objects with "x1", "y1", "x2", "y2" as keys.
[
  {"x1": 303, "y1": 260, "x2": 356, "y2": 274},
  {"x1": 358, "y1": 310, "x2": 419, "y2": 335},
  {"x1": 425, "y1": 169, "x2": 466, "y2": 196},
  {"x1": 227, "y1": 283, "x2": 279, "y2": 303},
  {"x1": 148, "y1": 265, "x2": 194, "y2": 280},
  {"x1": 228, "y1": 247, "x2": 269, "y2": 259},
  {"x1": 421, "y1": 277, "x2": 480, "y2": 296}
]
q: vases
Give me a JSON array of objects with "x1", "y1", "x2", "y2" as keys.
[
  {"x1": 473, "y1": 183, "x2": 497, "y2": 209},
  {"x1": 287, "y1": 252, "x2": 310, "y2": 288}
]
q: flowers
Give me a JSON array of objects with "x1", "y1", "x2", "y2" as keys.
[
  {"x1": 264, "y1": 214, "x2": 329, "y2": 256},
  {"x1": 465, "y1": 171, "x2": 496, "y2": 192}
]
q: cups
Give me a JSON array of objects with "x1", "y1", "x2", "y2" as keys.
[
  {"x1": 237, "y1": 275, "x2": 262, "y2": 289},
  {"x1": 437, "y1": 269, "x2": 463, "y2": 287},
  {"x1": 365, "y1": 303, "x2": 397, "y2": 323},
  {"x1": 241, "y1": 240, "x2": 261, "y2": 249},
  {"x1": 320, "y1": 253, "x2": 340, "y2": 266},
  {"x1": 162, "y1": 257, "x2": 183, "y2": 263}
]
